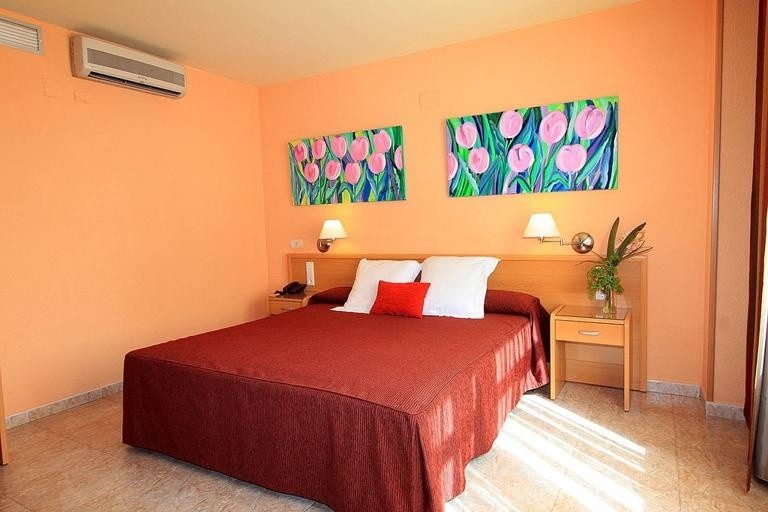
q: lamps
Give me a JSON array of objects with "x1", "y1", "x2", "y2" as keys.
[
  {"x1": 522, "y1": 212, "x2": 594, "y2": 254},
  {"x1": 317, "y1": 219, "x2": 347, "y2": 253}
]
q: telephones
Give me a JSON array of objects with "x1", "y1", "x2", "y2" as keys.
[{"x1": 274, "y1": 281, "x2": 307, "y2": 296}]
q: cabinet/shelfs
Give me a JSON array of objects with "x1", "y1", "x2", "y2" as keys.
[{"x1": 549, "y1": 303, "x2": 631, "y2": 411}]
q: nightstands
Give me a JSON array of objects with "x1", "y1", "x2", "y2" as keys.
[{"x1": 268, "y1": 291, "x2": 319, "y2": 316}]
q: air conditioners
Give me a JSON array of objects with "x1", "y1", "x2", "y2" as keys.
[{"x1": 70, "y1": 35, "x2": 186, "y2": 99}]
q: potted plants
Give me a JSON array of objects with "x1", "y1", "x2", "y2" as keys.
[{"x1": 576, "y1": 216, "x2": 654, "y2": 314}]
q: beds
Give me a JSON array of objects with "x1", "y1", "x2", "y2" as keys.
[{"x1": 123, "y1": 304, "x2": 539, "y2": 511}]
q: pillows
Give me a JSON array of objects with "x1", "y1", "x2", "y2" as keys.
[{"x1": 308, "y1": 256, "x2": 539, "y2": 317}]
